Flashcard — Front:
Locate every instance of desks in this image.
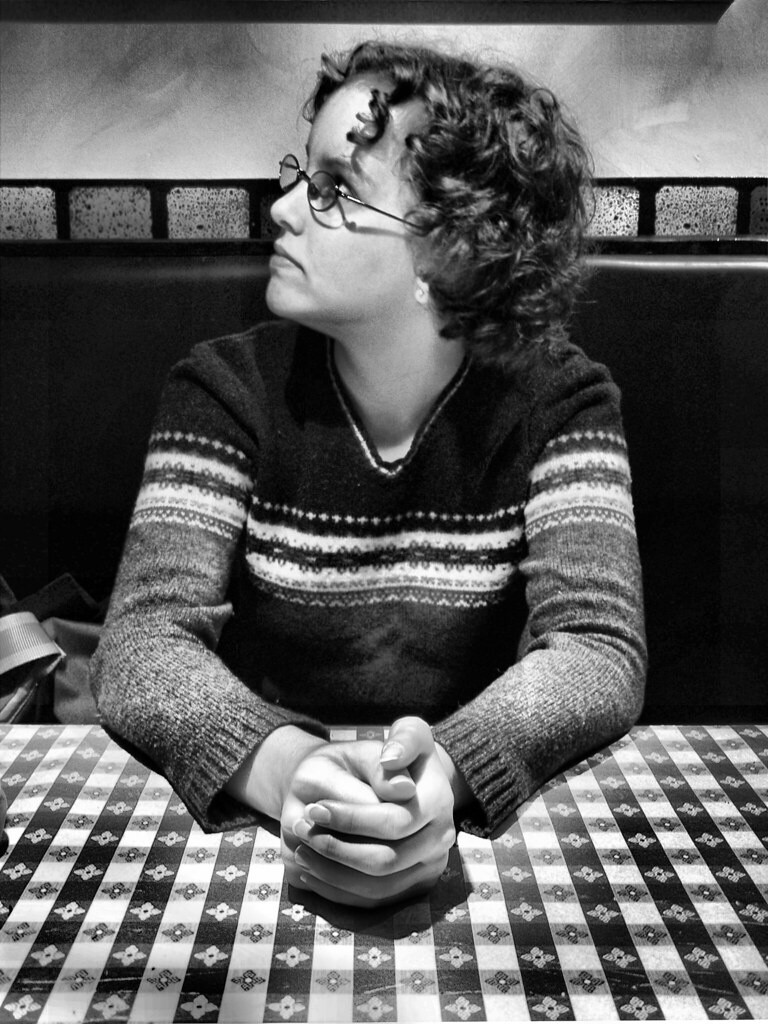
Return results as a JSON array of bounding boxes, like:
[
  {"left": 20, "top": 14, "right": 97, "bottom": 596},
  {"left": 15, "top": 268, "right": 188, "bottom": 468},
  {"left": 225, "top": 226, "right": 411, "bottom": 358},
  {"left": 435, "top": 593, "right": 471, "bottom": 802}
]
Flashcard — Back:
[{"left": 1, "top": 724, "right": 768, "bottom": 1021}]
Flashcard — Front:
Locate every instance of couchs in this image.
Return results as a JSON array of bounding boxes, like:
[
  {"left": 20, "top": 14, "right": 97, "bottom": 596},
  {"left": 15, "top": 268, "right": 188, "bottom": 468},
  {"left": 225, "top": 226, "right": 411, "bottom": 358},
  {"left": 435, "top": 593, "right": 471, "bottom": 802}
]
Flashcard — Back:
[{"left": 0, "top": 236, "right": 768, "bottom": 722}]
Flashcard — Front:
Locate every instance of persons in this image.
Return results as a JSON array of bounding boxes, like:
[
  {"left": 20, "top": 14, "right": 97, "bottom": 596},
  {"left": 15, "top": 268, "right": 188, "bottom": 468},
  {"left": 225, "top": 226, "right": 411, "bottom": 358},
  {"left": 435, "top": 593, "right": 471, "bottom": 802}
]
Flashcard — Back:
[{"left": 90, "top": 39, "right": 646, "bottom": 913}]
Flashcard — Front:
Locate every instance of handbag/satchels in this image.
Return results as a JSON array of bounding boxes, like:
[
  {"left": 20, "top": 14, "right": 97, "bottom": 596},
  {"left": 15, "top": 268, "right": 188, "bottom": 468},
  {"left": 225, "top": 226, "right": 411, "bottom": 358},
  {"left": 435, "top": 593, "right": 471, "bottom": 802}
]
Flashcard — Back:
[{"left": 0, "top": 570, "right": 106, "bottom": 725}]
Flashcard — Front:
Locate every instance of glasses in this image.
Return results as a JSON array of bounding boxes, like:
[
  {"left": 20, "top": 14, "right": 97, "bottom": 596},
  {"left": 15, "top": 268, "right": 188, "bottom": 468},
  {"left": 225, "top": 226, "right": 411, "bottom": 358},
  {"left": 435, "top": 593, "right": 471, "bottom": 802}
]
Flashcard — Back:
[{"left": 276, "top": 153, "right": 438, "bottom": 237}]
